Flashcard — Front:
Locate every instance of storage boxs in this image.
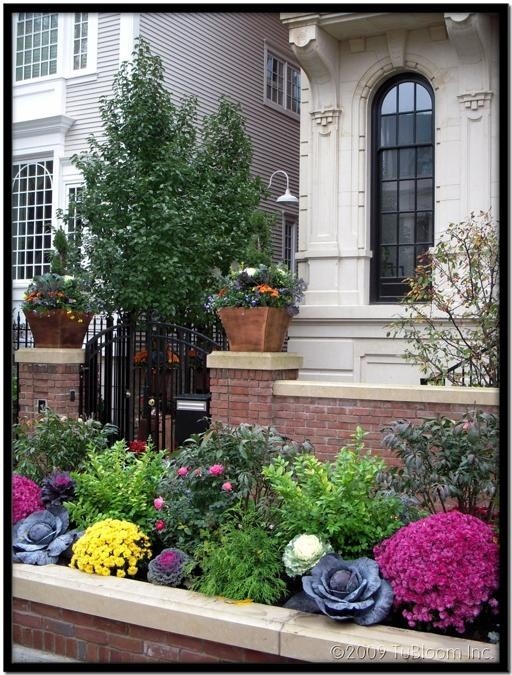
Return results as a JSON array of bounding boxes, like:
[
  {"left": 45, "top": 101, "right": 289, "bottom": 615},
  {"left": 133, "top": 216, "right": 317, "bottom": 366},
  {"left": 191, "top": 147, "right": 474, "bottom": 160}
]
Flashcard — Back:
[{"left": 173, "top": 393, "right": 211, "bottom": 447}]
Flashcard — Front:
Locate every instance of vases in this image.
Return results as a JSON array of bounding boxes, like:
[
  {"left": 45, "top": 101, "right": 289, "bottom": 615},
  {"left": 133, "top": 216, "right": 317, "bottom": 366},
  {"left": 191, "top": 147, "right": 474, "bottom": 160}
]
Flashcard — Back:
[
  {"left": 214, "top": 307, "right": 290, "bottom": 352},
  {"left": 23, "top": 308, "right": 94, "bottom": 348}
]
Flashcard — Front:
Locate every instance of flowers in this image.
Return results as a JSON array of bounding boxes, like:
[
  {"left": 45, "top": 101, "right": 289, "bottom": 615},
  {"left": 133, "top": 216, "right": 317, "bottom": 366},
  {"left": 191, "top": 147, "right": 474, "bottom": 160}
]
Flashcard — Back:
[
  {"left": 20, "top": 273, "right": 102, "bottom": 323},
  {"left": 203, "top": 259, "right": 310, "bottom": 317}
]
